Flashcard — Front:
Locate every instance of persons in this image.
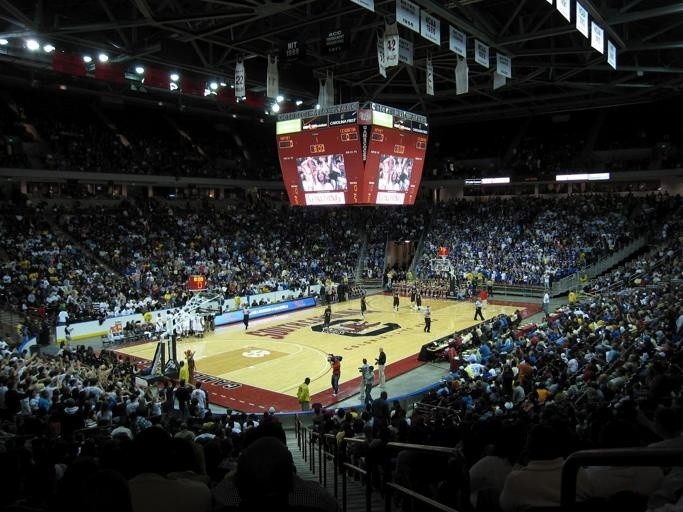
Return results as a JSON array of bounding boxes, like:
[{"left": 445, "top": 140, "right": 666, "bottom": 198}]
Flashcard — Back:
[{"left": 1, "top": 153, "right": 682, "bottom": 512}]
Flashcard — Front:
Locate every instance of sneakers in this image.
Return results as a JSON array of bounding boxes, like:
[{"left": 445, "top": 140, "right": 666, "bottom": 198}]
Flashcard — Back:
[
  {"left": 332, "top": 389, "right": 339, "bottom": 397},
  {"left": 378, "top": 384, "right": 385, "bottom": 388}
]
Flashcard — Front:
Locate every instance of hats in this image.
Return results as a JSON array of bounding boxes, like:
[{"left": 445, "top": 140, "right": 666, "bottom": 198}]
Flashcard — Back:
[{"left": 269, "top": 407, "right": 275, "bottom": 413}]
